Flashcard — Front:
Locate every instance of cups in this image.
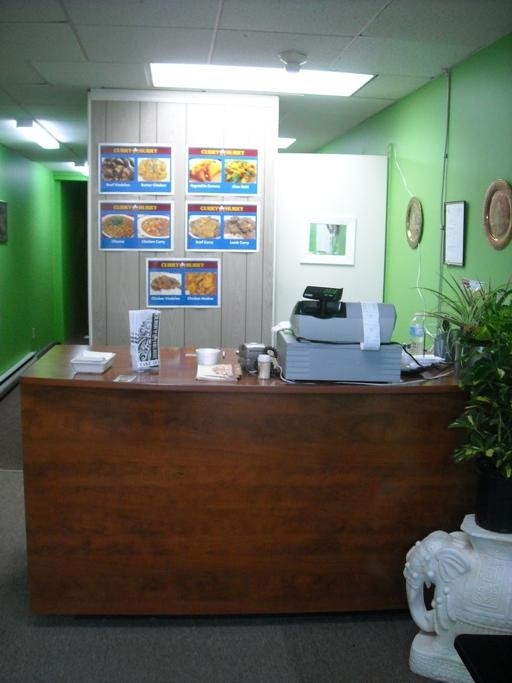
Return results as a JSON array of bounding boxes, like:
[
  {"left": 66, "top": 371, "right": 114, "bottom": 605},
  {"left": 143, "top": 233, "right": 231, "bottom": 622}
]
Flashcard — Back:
[
  {"left": 433, "top": 332, "right": 456, "bottom": 370},
  {"left": 195, "top": 347, "right": 221, "bottom": 364},
  {"left": 257, "top": 353, "right": 272, "bottom": 379}
]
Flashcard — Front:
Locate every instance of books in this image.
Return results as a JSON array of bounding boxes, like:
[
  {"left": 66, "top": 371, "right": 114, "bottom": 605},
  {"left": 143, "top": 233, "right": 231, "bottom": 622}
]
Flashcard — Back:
[{"left": 196, "top": 362, "right": 242, "bottom": 381}]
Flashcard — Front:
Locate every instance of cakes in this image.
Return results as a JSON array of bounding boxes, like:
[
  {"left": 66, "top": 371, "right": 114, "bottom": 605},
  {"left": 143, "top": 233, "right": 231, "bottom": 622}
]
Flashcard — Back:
[{"left": 190, "top": 217, "right": 220, "bottom": 239}]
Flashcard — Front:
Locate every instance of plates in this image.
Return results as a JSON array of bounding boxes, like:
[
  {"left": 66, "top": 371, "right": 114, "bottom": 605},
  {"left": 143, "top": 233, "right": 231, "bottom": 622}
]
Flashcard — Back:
[
  {"left": 188, "top": 215, "right": 222, "bottom": 240},
  {"left": 101, "top": 213, "right": 135, "bottom": 238},
  {"left": 405, "top": 196, "right": 423, "bottom": 248},
  {"left": 137, "top": 214, "right": 170, "bottom": 239},
  {"left": 482, "top": 178, "right": 512, "bottom": 248},
  {"left": 70, "top": 350, "right": 117, "bottom": 373}
]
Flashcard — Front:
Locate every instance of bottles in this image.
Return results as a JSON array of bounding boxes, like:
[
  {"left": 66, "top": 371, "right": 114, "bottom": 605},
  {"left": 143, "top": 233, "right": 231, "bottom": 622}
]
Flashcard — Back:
[{"left": 408, "top": 313, "right": 426, "bottom": 360}]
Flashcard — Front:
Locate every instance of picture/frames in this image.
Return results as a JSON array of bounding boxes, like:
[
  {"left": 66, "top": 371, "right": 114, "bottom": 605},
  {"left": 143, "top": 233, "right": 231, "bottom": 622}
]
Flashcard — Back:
[
  {"left": 0, "top": 201, "right": 9, "bottom": 243},
  {"left": 444, "top": 201, "right": 466, "bottom": 267},
  {"left": 299, "top": 215, "right": 357, "bottom": 266}
]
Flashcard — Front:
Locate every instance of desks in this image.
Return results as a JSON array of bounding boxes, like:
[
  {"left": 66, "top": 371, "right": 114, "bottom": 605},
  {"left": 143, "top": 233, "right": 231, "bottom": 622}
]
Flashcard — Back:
[{"left": 454, "top": 634, "right": 512, "bottom": 683}]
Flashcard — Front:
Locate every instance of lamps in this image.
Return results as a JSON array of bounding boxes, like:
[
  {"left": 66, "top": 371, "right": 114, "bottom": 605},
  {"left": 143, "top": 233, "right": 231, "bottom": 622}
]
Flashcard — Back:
[
  {"left": 278, "top": 51, "right": 311, "bottom": 75},
  {"left": 15, "top": 119, "right": 60, "bottom": 149}
]
